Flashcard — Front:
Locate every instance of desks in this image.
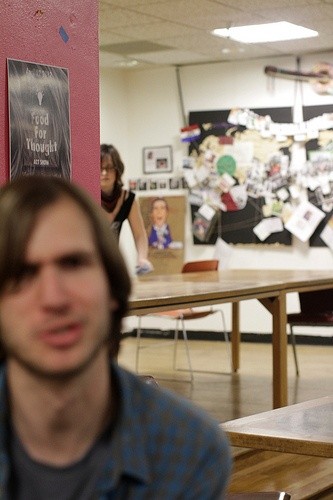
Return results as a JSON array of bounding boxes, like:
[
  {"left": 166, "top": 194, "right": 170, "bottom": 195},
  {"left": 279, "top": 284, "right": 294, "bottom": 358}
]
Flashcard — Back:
[
  {"left": 127, "top": 269, "right": 333, "bottom": 409},
  {"left": 218, "top": 395, "right": 333, "bottom": 459}
]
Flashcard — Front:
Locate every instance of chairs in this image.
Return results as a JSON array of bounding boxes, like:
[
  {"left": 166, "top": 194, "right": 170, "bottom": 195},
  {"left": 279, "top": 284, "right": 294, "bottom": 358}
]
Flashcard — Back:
[
  {"left": 135, "top": 259, "right": 236, "bottom": 383},
  {"left": 287, "top": 288, "right": 332, "bottom": 377}
]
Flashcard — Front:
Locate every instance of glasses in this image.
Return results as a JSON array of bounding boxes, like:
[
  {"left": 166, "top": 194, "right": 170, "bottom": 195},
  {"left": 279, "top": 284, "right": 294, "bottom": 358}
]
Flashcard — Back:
[{"left": 101, "top": 164, "right": 117, "bottom": 174}]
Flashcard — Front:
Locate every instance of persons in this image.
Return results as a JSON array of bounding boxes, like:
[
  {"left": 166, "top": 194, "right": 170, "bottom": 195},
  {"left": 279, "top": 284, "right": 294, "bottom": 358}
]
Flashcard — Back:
[
  {"left": 0, "top": 175, "right": 232, "bottom": 500},
  {"left": 147, "top": 198, "right": 183, "bottom": 249},
  {"left": 100, "top": 142, "right": 155, "bottom": 274}
]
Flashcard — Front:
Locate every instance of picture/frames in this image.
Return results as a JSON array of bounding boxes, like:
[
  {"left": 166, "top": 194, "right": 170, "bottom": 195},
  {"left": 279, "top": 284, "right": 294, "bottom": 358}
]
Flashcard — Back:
[{"left": 143, "top": 146, "right": 173, "bottom": 174}]
